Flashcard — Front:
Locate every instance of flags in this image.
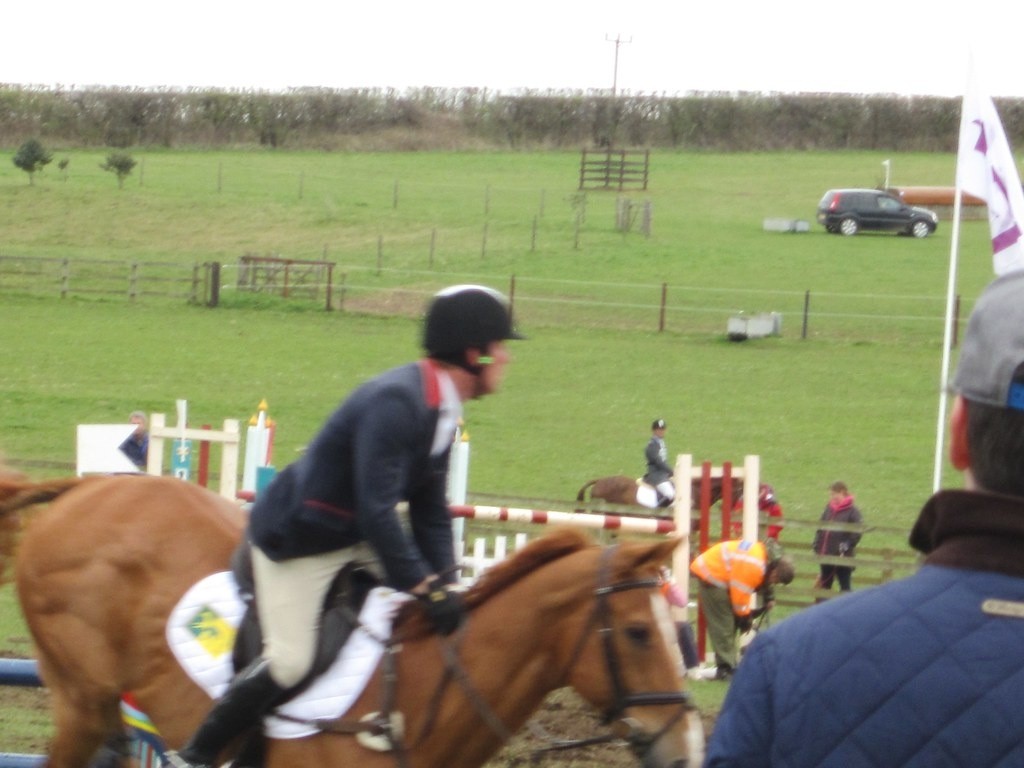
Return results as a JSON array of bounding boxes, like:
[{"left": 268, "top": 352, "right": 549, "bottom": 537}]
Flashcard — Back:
[{"left": 959, "top": 69, "right": 1024, "bottom": 277}]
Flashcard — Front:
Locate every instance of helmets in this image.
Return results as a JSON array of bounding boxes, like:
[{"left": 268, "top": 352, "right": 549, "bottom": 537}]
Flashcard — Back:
[
  {"left": 653, "top": 419, "right": 666, "bottom": 429},
  {"left": 421, "top": 286, "right": 526, "bottom": 376}
]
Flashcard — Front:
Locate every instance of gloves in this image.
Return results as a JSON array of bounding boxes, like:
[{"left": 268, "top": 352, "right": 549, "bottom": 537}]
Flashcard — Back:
[{"left": 419, "top": 578, "right": 461, "bottom": 636}]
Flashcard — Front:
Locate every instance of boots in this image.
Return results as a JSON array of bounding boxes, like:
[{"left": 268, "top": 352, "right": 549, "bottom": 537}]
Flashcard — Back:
[
  {"left": 166, "top": 657, "right": 291, "bottom": 768},
  {"left": 657, "top": 496, "right": 674, "bottom": 507}
]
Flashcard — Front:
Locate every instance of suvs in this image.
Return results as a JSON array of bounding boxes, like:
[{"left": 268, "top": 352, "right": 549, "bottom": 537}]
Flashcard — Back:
[{"left": 817, "top": 189, "right": 939, "bottom": 238}]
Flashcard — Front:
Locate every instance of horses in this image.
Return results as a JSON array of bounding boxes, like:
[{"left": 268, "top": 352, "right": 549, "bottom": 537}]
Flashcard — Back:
[
  {"left": 0, "top": 470, "right": 705, "bottom": 768},
  {"left": 577, "top": 476, "right": 744, "bottom": 527}
]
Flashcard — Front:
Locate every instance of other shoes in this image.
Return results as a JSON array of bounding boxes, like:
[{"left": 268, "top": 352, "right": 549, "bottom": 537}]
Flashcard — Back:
[{"left": 715, "top": 668, "right": 737, "bottom": 679}]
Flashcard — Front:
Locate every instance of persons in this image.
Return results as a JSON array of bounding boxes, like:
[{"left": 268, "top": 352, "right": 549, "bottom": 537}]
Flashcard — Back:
[
  {"left": 645, "top": 420, "right": 675, "bottom": 510},
  {"left": 160, "top": 284, "right": 524, "bottom": 768},
  {"left": 690, "top": 537, "right": 794, "bottom": 679},
  {"left": 812, "top": 481, "right": 865, "bottom": 603},
  {"left": 702, "top": 271, "right": 1023, "bottom": 768},
  {"left": 755, "top": 485, "right": 785, "bottom": 541}
]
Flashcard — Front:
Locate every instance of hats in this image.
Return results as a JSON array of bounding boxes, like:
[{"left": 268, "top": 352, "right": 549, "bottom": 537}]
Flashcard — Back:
[{"left": 947, "top": 268, "right": 1024, "bottom": 410}]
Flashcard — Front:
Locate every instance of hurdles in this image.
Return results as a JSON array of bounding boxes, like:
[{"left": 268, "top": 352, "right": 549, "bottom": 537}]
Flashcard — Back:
[
  {"left": 132, "top": 409, "right": 763, "bottom": 681},
  {"left": 234, "top": 396, "right": 695, "bottom": 624}
]
